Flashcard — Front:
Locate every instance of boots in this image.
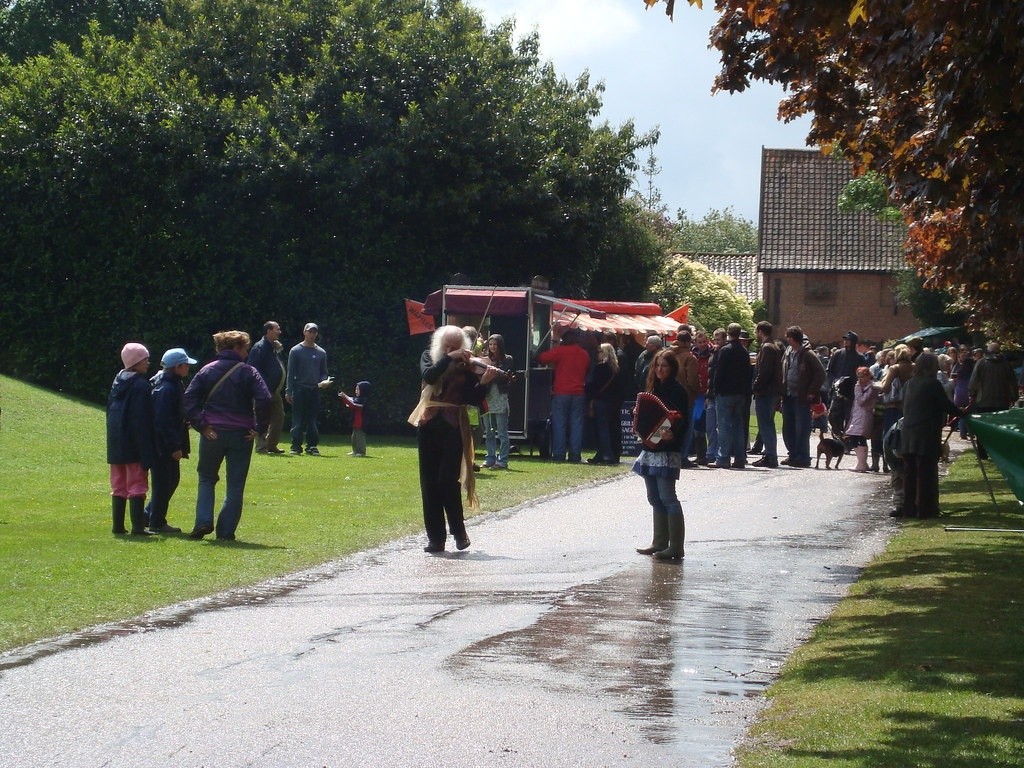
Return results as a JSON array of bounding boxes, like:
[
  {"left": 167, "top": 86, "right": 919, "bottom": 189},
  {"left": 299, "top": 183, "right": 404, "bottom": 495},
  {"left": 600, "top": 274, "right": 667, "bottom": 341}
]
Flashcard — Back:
[
  {"left": 636, "top": 511, "right": 670, "bottom": 556},
  {"left": 655, "top": 514, "right": 684, "bottom": 560},
  {"left": 127, "top": 494, "right": 154, "bottom": 535},
  {"left": 112, "top": 494, "right": 128, "bottom": 534},
  {"left": 848, "top": 447, "right": 870, "bottom": 473}
]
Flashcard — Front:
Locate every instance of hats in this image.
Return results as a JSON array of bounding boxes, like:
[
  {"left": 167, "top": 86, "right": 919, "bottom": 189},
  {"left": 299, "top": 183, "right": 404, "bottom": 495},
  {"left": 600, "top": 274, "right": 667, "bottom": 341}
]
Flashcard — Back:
[
  {"left": 161, "top": 347, "right": 198, "bottom": 367},
  {"left": 842, "top": 333, "right": 851, "bottom": 339},
  {"left": 304, "top": 323, "right": 318, "bottom": 332},
  {"left": 739, "top": 331, "right": 755, "bottom": 341},
  {"left": 121, "top": 343, "right": 150, "bottom": 370}
]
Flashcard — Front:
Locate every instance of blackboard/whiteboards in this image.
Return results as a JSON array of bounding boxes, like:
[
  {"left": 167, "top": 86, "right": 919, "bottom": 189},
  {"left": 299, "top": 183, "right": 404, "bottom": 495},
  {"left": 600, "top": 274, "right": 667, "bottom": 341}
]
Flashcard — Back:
[{"left": 619, "top": 402, "right": 644, "bottom": 457}]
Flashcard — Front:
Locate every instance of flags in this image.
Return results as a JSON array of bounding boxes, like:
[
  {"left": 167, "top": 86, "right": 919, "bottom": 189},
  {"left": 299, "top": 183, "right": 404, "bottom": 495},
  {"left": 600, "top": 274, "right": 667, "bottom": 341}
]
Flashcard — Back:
[
  {"left": 405, "top": 299, "right": 435, "bottom": 335},
  {"left": 665, "top": 305, "right": 688, "bottom": 341}
]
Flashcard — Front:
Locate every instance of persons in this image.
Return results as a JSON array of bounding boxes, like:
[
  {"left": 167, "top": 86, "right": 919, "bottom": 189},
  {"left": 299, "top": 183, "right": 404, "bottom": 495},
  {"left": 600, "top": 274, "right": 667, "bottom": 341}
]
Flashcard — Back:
[
  {"left": 462, "top": 326, "right": 480, "bottom": 472},
  {"left": 150, "top": 348, "right": 197, "bottom": 531},
  {"left": 185, "top": 332, "right": 272, "bottom": 542},
  {"left": 407, "top": 324, "right": 497, "bottom": 551},
  {"left": 480, "top": 334, "right": 513, "bottom": 469},
  {"left": 883, "top": 415, "right": 949, "bottom": 513},
  {"left": 903, "top": 352, "right": 968, "bottom": 511},
  {"left": 543, "top": 332, "right": 590, "bottom": 463},
  {"left": 601, "top": 332, "right": 625, "bottom": 366},
  {"left": 631, "top": 348, "right": 689, "bottom": 559},
  {"left": 285, "top": 323, "right": 328, "bottom": 456},
  {"left": 588, "top": 343, "right": 619, "bottom": 464},
  {"left": 338, "top": 380, "right": 371, "bottom": 457},
  {"left": 105, "top": 342, "right": 158, "bottom": 535},
  {"left": 624, "top": 320, "right": 1016, "bottom": 471},
  {"left": 249, "top": 321, "right": 285, "bottom": 453}
]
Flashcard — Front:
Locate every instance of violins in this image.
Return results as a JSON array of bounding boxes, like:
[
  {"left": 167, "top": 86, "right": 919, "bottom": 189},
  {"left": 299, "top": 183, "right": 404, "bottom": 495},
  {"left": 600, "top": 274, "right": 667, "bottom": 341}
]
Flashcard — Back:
[{"left": 460, "top": 353, "right": 518, "bottom": 384}]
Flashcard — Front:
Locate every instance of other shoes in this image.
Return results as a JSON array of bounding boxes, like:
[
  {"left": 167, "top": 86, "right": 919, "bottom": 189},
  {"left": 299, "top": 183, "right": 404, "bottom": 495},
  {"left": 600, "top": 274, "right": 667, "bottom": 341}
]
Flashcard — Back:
[
  {"left": 305, "top": 445, "right": 321, "bottom": 457},
  {"left": 479, "top": 463, "right": 494, "bottom": 469},
  {"left": 149, "top": 521, "right": 182, "bottom": 532},
  {"left": 424, "top": 539, "right": 444, "bottom": 551},
  {"left": 489, "top": 463, "right": 503, "bottom": 470},
  {"left": 891, "top": 506, "right": 909, "bottom": 517},
  {"left": 188, "top": 522, "right": 213, "bottom": 539},
  {"left": 682, "top": 445, "right": 812, "bottom": 468},
  {"left": 587, "top": 457, "right": 598, "bottom": 463},
  {"left": 455, "top": 530, "right": 470, "bottom": 549},
  {"left": 267, "top": 447, "right": 286, "bottom": 454},
  {"left": 290, "top": 449, "right": 299, "bottom": 455},
  {"left": 347, "top": 450, "right": 365, "bottom": 457}
]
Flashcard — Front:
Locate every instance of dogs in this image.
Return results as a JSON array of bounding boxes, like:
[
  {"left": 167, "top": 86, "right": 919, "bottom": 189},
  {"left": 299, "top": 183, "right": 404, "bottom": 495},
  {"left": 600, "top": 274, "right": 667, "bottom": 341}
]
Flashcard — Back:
[{"left": 815, "top": 427, "right": 850, "bottom": 469}]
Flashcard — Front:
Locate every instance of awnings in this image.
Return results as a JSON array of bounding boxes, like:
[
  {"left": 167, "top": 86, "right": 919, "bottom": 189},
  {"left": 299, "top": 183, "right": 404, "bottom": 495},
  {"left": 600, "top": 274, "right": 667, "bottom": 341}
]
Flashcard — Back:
[
  {"left": 553, "top": 315, "right": 696, "bottom": 336},
  {"left": 900, "top": 326, "right": 959, "bottom": 343}
]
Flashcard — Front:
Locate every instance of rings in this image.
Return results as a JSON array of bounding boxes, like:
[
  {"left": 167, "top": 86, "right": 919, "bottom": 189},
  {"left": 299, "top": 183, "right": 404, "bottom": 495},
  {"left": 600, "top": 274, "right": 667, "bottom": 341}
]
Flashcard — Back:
[{"left": 210, "top": 432, "right": 212, "bottom": 434}]
